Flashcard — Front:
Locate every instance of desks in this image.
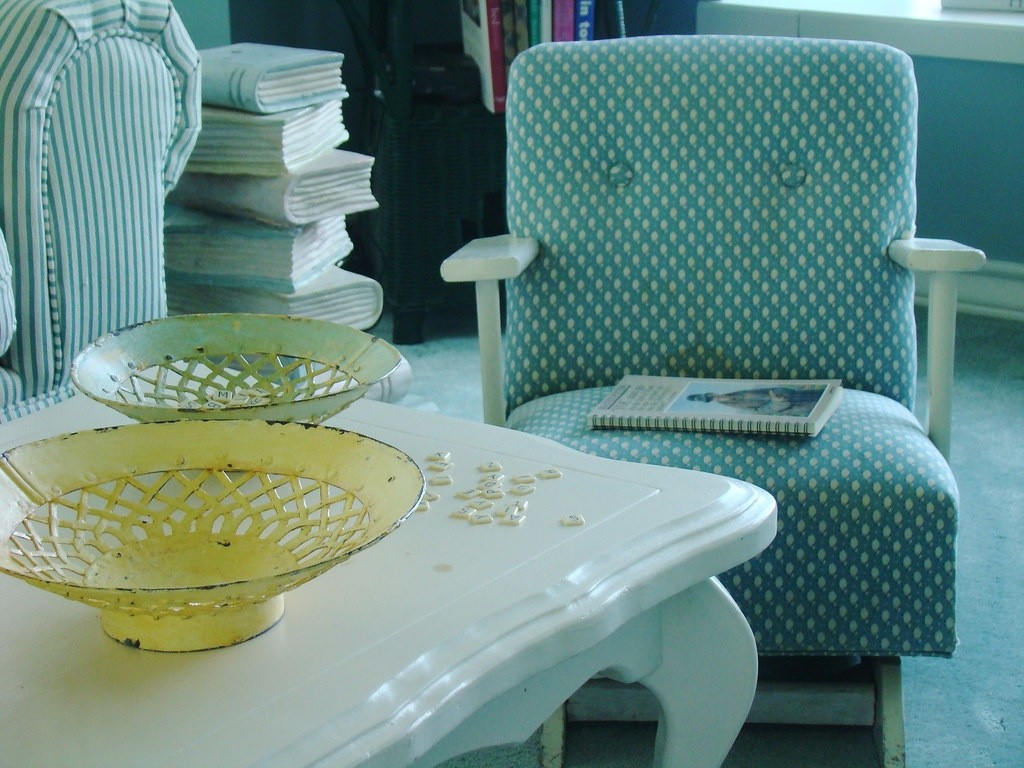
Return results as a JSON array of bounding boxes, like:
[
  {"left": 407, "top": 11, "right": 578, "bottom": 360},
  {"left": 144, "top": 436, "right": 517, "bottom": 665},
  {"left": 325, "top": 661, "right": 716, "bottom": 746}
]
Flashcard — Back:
[{"left": 0, "top": 360, "right": 779, "bottom": 768}]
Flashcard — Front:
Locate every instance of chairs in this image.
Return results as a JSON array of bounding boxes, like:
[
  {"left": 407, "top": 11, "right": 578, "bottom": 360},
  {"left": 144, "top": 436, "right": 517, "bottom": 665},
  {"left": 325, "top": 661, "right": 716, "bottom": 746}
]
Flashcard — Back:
[{"left": 440, "top": 34, "right": 987, "bottom": 767}]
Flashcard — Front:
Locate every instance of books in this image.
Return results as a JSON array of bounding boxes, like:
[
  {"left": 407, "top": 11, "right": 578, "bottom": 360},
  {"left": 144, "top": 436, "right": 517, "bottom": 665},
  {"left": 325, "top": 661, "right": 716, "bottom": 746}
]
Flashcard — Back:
[
  {"left": 461, "top": 0, "right": 595, "bottom": 114},
  {"left": 587, "top": 375, "right": 844, "bottom": 437},
  {"left": 161, "top": 42, "right": 435, "bottom": 410},
  {"left": 941, "top": 1, "right": 1024, "bottom": 12}
]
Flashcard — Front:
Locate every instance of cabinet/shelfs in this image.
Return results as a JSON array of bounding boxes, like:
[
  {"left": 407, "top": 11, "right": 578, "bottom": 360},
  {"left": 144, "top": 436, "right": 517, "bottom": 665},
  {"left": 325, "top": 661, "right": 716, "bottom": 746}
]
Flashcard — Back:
[{"left": 694, "top": 0, "right": 1024, "bottom": 320}]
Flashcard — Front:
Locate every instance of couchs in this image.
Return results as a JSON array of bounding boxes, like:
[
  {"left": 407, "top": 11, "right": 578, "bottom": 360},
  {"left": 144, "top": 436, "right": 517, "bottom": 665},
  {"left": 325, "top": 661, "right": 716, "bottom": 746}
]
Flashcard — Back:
[{"left": 0, "top": 0, "right": 203, "bottom": 423}]
